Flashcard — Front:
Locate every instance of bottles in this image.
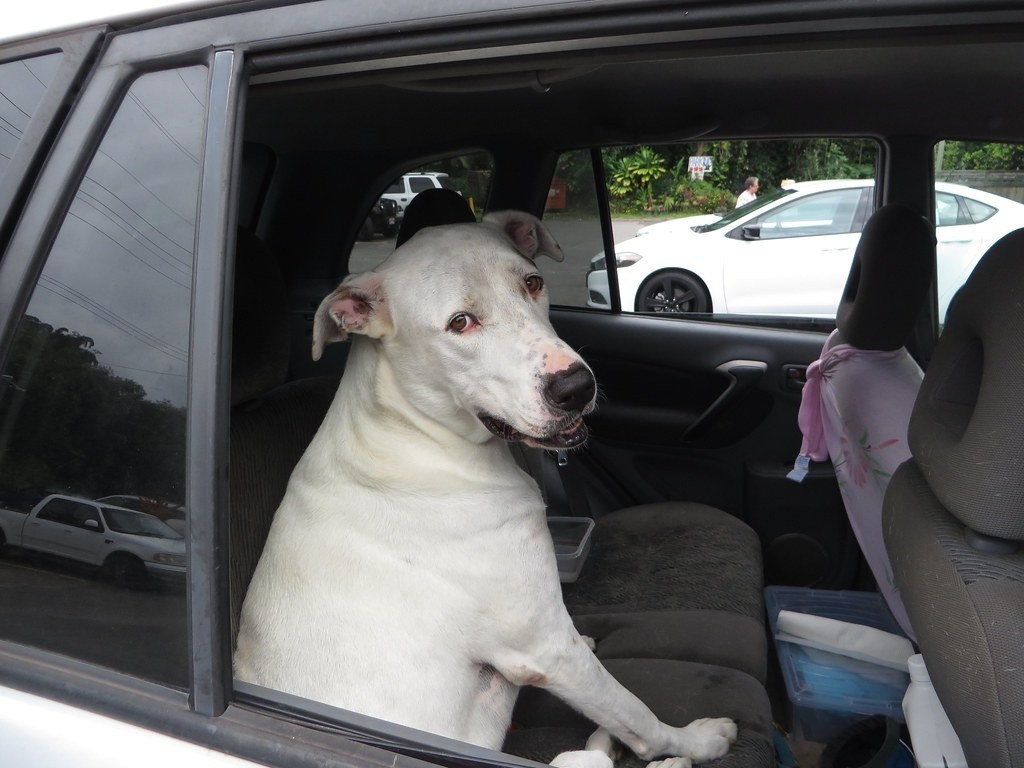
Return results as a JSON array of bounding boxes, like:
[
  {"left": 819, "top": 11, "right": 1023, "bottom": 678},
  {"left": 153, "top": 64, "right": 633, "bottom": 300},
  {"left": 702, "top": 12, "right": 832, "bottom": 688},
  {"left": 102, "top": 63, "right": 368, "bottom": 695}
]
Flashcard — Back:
[{"left": 902, "top": 654, "right": 969, "bottom": 768}]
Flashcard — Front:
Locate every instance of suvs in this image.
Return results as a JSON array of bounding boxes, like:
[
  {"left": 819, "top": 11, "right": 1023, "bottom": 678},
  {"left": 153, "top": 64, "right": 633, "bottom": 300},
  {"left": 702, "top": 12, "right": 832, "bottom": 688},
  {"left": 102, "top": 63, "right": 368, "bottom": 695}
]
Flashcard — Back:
[
  {"left": 355, "top": 170, "right": 466, "bottom": 243},
  {"left": 0, "top": 1, "right": 1024, "bottom": 768}
]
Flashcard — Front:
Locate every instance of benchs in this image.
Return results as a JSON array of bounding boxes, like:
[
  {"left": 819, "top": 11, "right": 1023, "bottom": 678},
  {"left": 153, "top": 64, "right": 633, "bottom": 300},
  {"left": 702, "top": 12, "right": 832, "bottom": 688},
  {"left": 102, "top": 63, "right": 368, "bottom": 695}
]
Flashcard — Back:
[{"left": 230, "top": 191, "right": 774, "bottom": 768}]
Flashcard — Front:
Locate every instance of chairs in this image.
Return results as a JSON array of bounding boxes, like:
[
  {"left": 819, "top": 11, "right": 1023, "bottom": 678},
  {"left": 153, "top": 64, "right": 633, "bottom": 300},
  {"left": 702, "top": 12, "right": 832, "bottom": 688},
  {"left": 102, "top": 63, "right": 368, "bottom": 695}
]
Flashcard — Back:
[
  {"left": 882, "top": 227, "right": 1024, "bottom": 768},
  {"left": 796, "top": 205, "right": 941, "bottom": 648}
]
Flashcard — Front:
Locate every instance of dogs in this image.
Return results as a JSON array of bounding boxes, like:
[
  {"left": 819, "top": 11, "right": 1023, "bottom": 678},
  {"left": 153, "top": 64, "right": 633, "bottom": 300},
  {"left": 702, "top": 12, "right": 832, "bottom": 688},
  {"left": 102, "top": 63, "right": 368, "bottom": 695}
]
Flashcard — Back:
[{"left": 230, "top": 211, "right": 738, "bottom": 768}]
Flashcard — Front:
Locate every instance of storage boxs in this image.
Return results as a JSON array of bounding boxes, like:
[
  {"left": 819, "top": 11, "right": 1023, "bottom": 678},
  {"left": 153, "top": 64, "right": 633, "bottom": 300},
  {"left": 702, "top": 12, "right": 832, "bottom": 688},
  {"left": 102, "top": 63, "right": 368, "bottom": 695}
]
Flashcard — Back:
[{"left": 761, "top": 585, "right": 921, "bottom": 742}]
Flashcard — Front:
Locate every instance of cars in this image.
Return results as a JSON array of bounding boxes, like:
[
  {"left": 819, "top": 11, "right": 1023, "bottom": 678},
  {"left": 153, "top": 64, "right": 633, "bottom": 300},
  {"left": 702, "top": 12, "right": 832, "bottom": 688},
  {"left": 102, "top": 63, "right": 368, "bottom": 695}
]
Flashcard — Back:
[{"left": 586, "top": 178, "right": 1024, "bottom": 337}]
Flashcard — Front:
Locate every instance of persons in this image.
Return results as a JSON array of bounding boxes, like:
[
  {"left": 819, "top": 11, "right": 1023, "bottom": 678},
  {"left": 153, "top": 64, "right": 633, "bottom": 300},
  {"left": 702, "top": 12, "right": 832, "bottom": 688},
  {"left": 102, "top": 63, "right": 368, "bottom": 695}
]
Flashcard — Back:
[{"left": 735, "top": 177, "right": 759, "bottom": 209}]
